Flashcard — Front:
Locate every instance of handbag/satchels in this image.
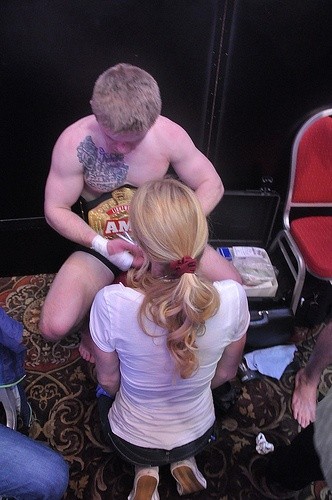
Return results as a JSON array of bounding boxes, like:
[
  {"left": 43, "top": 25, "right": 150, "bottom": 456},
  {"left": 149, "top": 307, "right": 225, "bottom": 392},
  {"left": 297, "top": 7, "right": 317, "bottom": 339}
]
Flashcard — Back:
[{"left": 278, "top": 280, "right": 332, "bottom": 328}]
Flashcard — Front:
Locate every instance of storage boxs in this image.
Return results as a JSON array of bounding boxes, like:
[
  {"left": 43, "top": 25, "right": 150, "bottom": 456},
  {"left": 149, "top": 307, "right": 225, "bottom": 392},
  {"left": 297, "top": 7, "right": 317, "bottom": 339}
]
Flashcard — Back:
[{"left": 202, "top": 187, "right": 295, "bottom": 351}]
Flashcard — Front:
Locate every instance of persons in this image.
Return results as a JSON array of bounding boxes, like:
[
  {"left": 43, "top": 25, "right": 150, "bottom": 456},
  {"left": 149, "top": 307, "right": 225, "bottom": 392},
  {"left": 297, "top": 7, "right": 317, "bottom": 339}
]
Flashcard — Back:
[
  {"left": 0, "top": 424, "right": 69, "bottom": 500},
  {"left": 89, "top": 178, "right": 251, "bottom": 500},
  {"left": 38, "top": 63, "right": 244, "bottom": 363},
  {"left": 265, "top": 320, "right": 332, "bottom": 493}
]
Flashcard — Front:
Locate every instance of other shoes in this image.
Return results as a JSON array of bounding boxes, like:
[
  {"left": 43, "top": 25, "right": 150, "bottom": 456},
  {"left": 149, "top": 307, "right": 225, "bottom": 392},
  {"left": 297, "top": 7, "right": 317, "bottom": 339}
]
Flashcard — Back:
[
  {"left": 238, "top": 445, "right": 271, "bottom": 496},
  {"left": 214, "top": 380, "right": 234, "bottom": 413}
]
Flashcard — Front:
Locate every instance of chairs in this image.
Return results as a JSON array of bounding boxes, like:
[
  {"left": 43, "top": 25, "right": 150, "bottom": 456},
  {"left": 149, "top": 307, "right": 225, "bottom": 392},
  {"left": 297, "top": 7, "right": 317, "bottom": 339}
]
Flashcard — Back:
[{"left": 269, "top": 109, "right": 332, "bottom": 318}]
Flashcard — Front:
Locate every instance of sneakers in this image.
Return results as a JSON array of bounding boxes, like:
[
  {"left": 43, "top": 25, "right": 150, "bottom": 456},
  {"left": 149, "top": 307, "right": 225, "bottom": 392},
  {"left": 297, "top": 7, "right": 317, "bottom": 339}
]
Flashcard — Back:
[
  {"left": 127, "top": 464, "right": 160, "bottom": 500},
  {"left": 170, "top": 456, "right": 207, "bottom": 495}
]
